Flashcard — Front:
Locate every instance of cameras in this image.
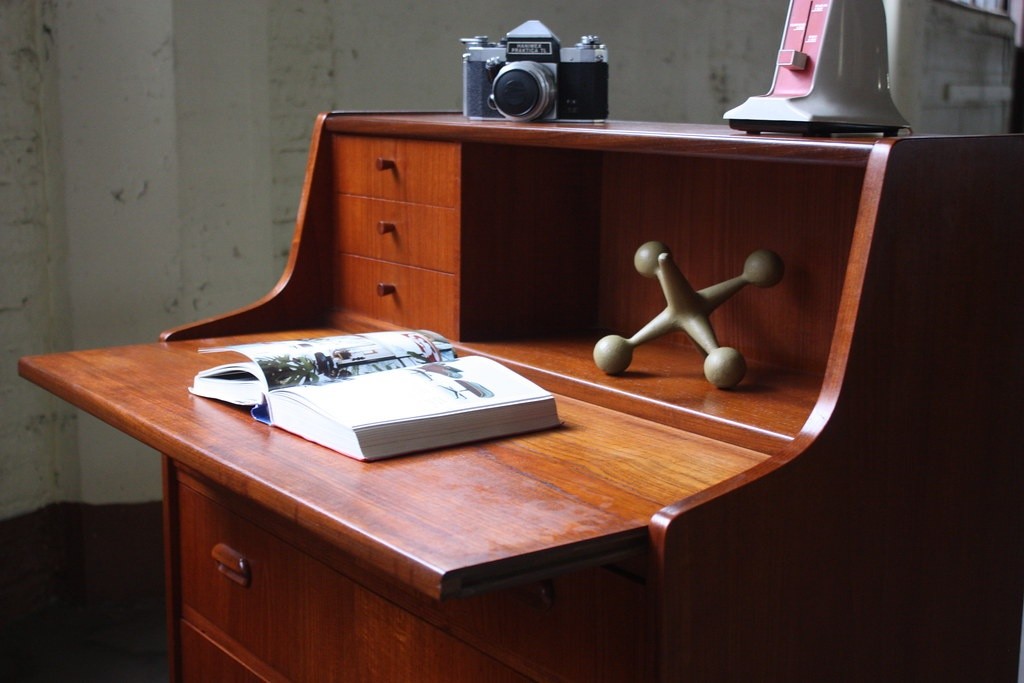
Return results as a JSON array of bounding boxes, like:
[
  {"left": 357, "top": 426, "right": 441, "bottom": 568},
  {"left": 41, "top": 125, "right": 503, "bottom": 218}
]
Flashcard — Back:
[{"left": 460, "top": 20, "right": 609, "bottom": 123}]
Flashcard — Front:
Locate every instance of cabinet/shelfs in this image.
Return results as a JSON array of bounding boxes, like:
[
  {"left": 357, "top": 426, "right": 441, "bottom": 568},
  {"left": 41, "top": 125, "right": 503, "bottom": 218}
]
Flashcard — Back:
[{"left": 16, "top": 106, "right": 1024, "bottom": 683}]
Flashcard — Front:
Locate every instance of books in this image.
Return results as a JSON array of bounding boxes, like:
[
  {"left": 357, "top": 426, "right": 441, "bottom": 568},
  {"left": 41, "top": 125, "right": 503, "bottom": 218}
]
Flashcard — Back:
[{"left": 188, "top": 330, "right": 565, "bottom": 462}]
